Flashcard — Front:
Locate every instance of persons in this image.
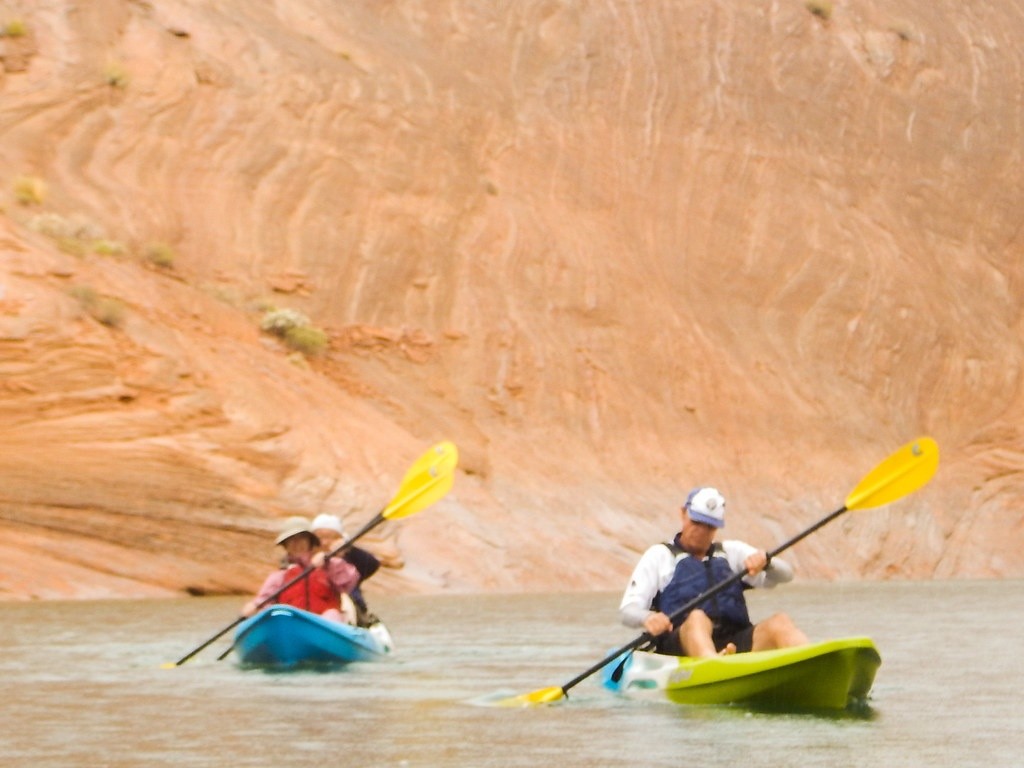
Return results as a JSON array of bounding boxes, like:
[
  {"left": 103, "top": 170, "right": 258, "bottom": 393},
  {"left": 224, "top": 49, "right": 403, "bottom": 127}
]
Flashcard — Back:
[
  {"left": 618, "top": 486, "right": 806, "bottom": 659},
  {"left": 237, "top": 510, "right": 406, "bottom": 629}
]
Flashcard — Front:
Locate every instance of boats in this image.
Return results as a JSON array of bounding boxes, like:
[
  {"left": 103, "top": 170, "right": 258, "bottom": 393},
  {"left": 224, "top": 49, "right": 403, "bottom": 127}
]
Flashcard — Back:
[
  {"left": 230, "top": 602, "right": 384, "bottom": 674},
  {"left": 602, "top": 635, "right": 884, "bottom": 715}
]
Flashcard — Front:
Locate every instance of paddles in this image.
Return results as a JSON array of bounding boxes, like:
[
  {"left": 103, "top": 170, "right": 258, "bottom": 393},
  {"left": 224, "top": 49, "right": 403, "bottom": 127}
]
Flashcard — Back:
[
  {"left": 499, "top": 435, "right": 940, "bottom": 711},
  {"left": 168, "top": 438, "right": 460, "bottom": 674}
]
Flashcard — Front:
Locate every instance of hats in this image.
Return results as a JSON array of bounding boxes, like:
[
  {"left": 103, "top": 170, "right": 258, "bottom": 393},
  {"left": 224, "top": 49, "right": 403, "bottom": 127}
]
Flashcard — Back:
[
  {"left": 686, "top": 487, "right": 725, "bottom": 528},
  {"left": 311, "top": 514, "right": 351, "bottom": 542},
  {"left": 274, "top": 516, "right": 321, "bottom": 547}
]
